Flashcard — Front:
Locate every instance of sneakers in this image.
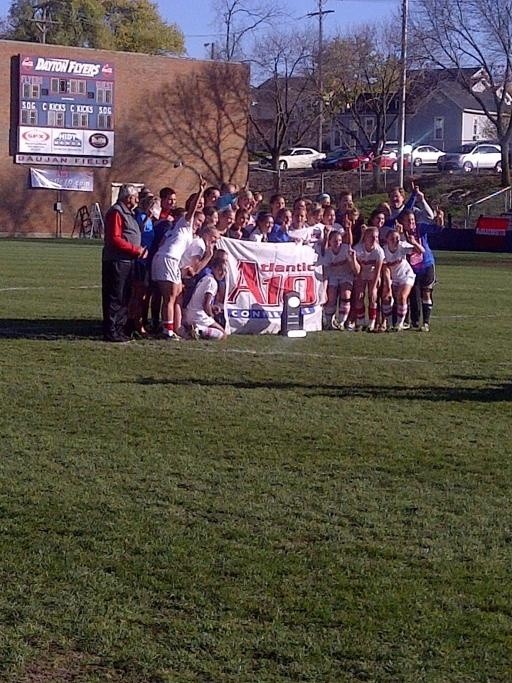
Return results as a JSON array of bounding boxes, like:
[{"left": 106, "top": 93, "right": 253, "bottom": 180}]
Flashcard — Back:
[
  {"left": 325, "top": 321, "right": 430, "bottom": 333},
  {"left": 192, "top": 321, "right": 200, "bottom": 339},
  {"left": 168, "top": 333, "right": 182, "bottom": 342}
]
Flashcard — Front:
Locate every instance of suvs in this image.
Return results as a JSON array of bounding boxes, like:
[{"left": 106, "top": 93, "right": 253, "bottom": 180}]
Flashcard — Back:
[
  {"left": 437, "top": 140, "right": 502, "bottom": 173},
  {"left": 380, "top": 140, "right": 411, "bottom": 163}
]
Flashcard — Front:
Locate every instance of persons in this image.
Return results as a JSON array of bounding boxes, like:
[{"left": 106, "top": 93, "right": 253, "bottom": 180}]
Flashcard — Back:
[{"left": 101, "top": 168, "right": 444, "bottom": 342}]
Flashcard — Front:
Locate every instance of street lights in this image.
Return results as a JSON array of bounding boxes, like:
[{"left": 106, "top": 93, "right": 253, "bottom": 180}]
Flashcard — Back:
[{"left": 204, "top": 39, "right": 215, "bottom": 59}]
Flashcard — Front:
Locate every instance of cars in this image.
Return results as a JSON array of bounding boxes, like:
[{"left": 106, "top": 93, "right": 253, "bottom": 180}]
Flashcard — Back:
[
  {"left": 318, "top": 145, "right": 351, "bottom": 168},
  {"left": 258, "top": 146, "right": 326, "bottom": 170},
  {"left": 409, "top": 144, "right": 447, "bottom": 166},
  {"left": 364, "top": 140, "right": 377, "bottom": 152},
  {"left": 336, "top": 144, "right": 408, "bottom": 172}
]
